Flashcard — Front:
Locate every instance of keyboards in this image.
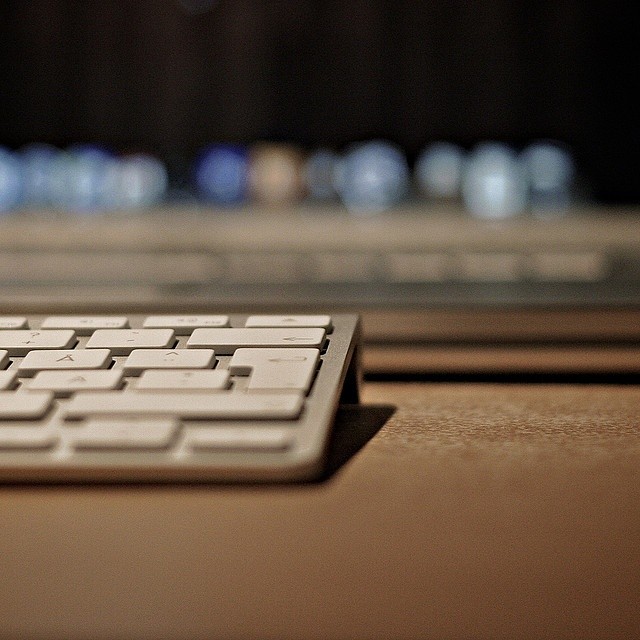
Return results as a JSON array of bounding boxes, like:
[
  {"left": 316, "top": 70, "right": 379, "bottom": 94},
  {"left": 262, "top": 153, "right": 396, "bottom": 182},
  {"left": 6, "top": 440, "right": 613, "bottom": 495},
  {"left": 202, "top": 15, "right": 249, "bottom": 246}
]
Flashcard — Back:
[{"left": 0, "top": 311, "right": 362, "bottom": 485}]
